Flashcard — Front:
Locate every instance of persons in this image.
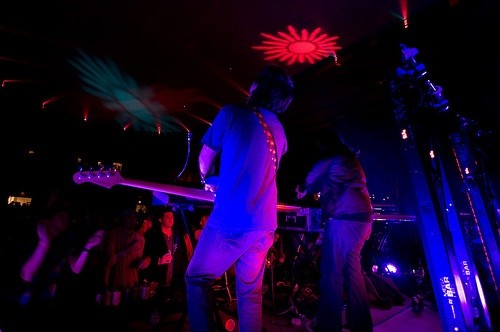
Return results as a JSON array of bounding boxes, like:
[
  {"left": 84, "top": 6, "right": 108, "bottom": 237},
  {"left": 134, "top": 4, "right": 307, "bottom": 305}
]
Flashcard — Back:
[
  {"left": 19, "top": 204, "right": 183, "bottom": 306},
  {"left": 185, "top": 66, "right": 374, "bottom": 332}
]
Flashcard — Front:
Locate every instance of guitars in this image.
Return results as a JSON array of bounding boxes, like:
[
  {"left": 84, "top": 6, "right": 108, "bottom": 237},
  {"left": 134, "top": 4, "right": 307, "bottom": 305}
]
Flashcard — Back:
[{"left": 71, "top": 161, "right": 215, "bottom": 203}]
